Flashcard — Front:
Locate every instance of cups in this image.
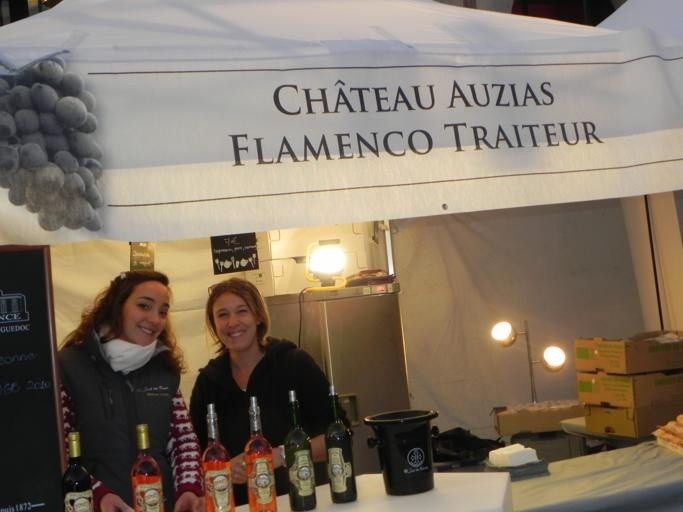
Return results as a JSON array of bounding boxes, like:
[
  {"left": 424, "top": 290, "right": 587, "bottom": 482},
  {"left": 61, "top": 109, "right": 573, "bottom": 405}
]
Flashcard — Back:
[{"left": 363, "top": 408, "right": 439, "bottom": 495}]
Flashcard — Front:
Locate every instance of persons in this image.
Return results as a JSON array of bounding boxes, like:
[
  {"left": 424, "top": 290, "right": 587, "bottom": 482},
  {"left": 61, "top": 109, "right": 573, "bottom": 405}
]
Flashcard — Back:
[
  {"left": 189, "top": 277, "right": 353, "bottom": 507},
  {"left": 56, "top": 268, "right": 205, "bottom": 511}
]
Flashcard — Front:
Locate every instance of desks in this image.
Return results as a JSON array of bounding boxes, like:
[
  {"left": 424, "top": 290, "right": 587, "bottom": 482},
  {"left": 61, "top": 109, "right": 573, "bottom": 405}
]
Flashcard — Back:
[
  {"left": 235, "top": 471, "right": 510, "bottom": 512},
  {"left": 561, "top": 416, "right": 607, "bottom": 458},
  {"left": 510, "top": 441, "right": 683, "bottom": 512}
]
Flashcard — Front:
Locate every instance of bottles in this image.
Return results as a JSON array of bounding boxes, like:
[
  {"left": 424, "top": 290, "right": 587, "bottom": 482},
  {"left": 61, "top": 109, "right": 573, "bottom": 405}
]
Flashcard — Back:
[
  {"left": 202, "top": 403, "right": 236, "bottom": 511},
  {"left": 325, "top": 385, "right": 358, "bottom": 503},
  {"left": 244, "top": 397, "right": 278, "bottom": 512},
  {"left": 131, "top": 422, "right": 164, "bottom": 512},
  {"left": 62, "top": 428, "right": 93, "bottom": 512},
  {"left": 283, "top": 389, "right": 316, "bottom": 512}
]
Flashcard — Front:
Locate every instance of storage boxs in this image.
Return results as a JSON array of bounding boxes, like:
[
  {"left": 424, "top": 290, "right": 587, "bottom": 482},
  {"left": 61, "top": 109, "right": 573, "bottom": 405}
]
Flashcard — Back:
[{"left": 574, "top": 330, "right": 683, "bottom": 438}]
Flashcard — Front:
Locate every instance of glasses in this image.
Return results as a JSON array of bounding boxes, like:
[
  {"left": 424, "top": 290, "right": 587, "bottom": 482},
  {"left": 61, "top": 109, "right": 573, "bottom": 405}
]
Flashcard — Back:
[{"left": 207, "top": 280, "right": 250, "bottom": 297}]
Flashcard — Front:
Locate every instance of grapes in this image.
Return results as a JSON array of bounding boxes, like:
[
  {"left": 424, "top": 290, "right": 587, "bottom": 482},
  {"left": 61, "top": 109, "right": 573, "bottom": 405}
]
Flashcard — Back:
[{"left": 0, "top": 51, "right": 104, "bottom": 233}]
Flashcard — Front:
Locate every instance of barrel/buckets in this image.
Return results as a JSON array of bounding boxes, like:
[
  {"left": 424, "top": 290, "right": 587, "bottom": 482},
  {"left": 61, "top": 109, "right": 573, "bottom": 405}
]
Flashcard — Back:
[{"left": 364, "top": 395, "right": 441, "bottom": 498}]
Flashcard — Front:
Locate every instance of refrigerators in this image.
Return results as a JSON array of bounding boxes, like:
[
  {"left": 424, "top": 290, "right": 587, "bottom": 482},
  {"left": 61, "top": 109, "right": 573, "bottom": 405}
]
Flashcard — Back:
[{"left": 263, "top": 282, "right": 410, "bottom": 476}]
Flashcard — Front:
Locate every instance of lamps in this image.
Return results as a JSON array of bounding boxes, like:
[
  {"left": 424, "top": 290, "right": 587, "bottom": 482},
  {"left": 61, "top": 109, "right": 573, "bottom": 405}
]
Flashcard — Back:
[
  {"left": 306, "top": 239, "right": 347, "bottom": 293},
  {"left": 491, "top": 320, "right": 566, "bottom": 404}
]
